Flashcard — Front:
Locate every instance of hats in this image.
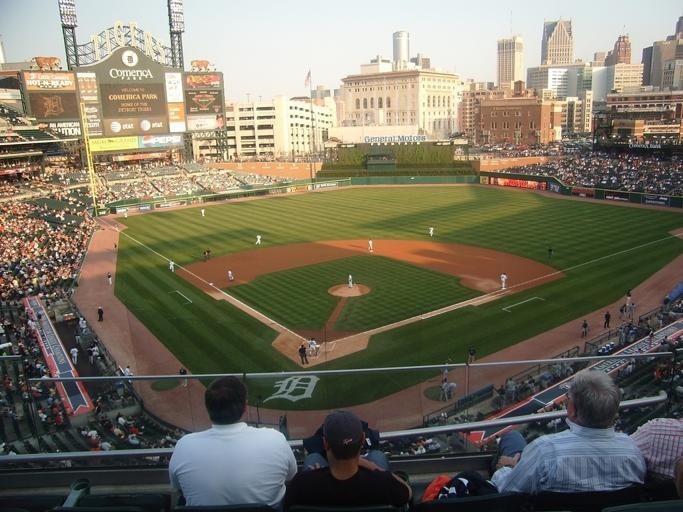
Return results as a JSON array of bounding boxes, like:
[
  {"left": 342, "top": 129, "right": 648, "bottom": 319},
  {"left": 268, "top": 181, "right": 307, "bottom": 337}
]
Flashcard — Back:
[{"left": 323, "top": 410, "right": 364, "bottom": 446}]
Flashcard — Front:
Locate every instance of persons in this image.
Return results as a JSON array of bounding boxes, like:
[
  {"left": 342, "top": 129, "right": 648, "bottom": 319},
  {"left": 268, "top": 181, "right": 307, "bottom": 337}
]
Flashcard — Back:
[
  {"left": 255, "top": 234, "right": 261, "bottom": 245},
  {"left": 202, "top": 248, "right": 210, "bottom": 257},
  {"left": 367, "top": 239, "right": 372, "bottom": 250},
  {"left": 168, "top": 375, "right": 299, "bottom": 512},
  {"left": 298, "top": 344, "right": 309, "bottom": 365},
  {"left": 460, "top": 126, "right": 682, "bottom": 201},
  {"left": 348, "top": 273, "right": 353, "bottom": 287},
  {"left": 548, "top": 247, "right": 552, "bottom": 257},
  {"left": 308, "top": 337, "right": 318, "bottom": 356},
  {"left": 200, "top": 208, "right": 205, "bottom": 217},
  {"left": 0, "top": 101, "right": 66, "bottom": 150},
  {"left": 500, "top": 272, "right": 507, "bottom": 289},
  {"left": 283, "top": 408, "right": 416, "bottom": 512},
  {"left": 428, "top": 226, "right": 433, "bottom": 236},
  {"left": 227, "top": 270, "right": 233, "bottom": 281},
  {"left": 288, "top": 434, "right": 424, "bottom": 466},
  {"left": 625, "top": 289, "right": 632, "bottom": 301}
]
